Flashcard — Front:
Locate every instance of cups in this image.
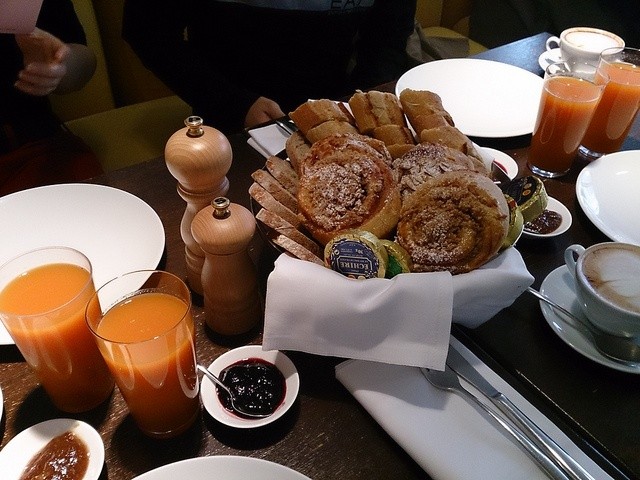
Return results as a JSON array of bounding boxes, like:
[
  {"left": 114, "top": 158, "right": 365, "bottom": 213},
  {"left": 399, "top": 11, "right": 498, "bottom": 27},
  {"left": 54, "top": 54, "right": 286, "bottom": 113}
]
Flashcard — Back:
[
  {"left": 577, "top": 47, "right": 640, "bottom": 159},
  {"left": 565, "top": 242, "right": 640, "bottom": 339},
  {"left": 85, "top": 269, "right": 201, "bottom": 439},
  {"left": 1, "top": 247, "right": 115, "bottom": 414},
  {"left": 526, "top": 62, "right": 608, "bottom": 177},
  {"left": 546, "top": 27, "right": 625, "bottom": 74}
]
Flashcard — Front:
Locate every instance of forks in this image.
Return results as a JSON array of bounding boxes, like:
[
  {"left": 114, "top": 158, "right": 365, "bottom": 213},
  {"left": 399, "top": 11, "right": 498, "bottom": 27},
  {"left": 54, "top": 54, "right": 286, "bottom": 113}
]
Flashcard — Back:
[{"left": 419, "top": 363, "right": 570, "bottom": 479}]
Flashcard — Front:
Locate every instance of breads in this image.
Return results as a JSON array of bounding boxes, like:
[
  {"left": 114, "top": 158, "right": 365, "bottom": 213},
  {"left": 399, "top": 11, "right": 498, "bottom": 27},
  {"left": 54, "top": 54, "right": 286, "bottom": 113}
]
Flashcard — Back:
[
  {"left": 392, "top": 140, "right": 474, "bottom": 206},
  {"left": 386, "top": 145, "right": 415, "bottom": 160},
  {"left": 421, "top": 127, "right": 472, "bottom": 154},
  {"left": 300, "top": 133, "right": 392, "bottom": 176},
  {"left": 288, "top": 99, "right": 355, "bottom": 136},
  {"left": 248, "top": 182, "right": 311, "bottom": 229},
  {"left": 306, "top": 120, "right": 359, "bottom": 144},
  {"left": 373, "top": 125, "right": 414, "bottom": 146},
  {"left": 298, "top": 150, "right": 401, "bottom": 246},
  {"left": 275, "top": 235, "right": 324, "bottom": 268},
  {"left": 265, "top": 155, "right": 315, "bottom": 195},
  {"left": 347, "top": 89, "right": 408, "bottom": 132},
  {"left": 285, "top": 129, "right": 310, "bottom": 174},
  {"left": 256, "top": 208, "right": 332, "bottom": 259},
  {"left": 397, "top": 170, "right": 511, "bottom": 274},
  {"left": 251, "top": 168, "right": 308, "bottom": 214},
  {"left": 399, "top": 88, "right": 455, "bottom": 139}
]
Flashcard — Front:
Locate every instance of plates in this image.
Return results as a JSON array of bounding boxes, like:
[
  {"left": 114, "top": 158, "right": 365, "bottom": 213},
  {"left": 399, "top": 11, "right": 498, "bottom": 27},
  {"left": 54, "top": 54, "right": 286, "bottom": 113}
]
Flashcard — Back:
[
  {"left": 202, "top": 344, "right": 300, "bottom": 430},
  {"left": 1, "top": 419, "right": 105, "bottom": 479},
  {"left": 539, "top": 262, "right": 640, "bottom": 374},
  {"left": 132, "top": 455, "right": 312, "bottom": 480},
  {"left": 1, "top": 184, "right": 165, "bottom": 346},
  {"left": 471, "top": 146, "right": 520, "bottom": 184},
  {"left": 522, "top": 196, "right": 573, "bottom": 237},
  {"left": 396, "top": 57, "right": 544, "bottom": 138},
  {"left": 576, "top": 149, "right": 640, "bottom": 245}
]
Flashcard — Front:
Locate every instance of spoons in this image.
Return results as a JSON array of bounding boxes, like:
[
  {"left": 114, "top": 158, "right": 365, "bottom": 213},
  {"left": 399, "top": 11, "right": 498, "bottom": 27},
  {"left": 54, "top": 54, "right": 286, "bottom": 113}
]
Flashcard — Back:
[
  {"left": 197, "top": 361, "right": 272, "bottom": 419},
  {"left": 527, "top": 287, "right": 640, "bottom": 363}
]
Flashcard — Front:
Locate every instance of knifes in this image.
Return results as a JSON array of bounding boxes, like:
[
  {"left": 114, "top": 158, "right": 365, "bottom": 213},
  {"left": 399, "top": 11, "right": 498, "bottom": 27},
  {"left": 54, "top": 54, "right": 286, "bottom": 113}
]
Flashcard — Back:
[{"left": 446, "top": 343, "right": 597, "bottom": 479}]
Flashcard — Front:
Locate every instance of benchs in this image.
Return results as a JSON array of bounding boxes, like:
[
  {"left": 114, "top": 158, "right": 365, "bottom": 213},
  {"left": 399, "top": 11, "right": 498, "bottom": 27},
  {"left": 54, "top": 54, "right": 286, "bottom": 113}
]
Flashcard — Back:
[{"left": 27, "top": 0, "right": 490, "bottom": 181}]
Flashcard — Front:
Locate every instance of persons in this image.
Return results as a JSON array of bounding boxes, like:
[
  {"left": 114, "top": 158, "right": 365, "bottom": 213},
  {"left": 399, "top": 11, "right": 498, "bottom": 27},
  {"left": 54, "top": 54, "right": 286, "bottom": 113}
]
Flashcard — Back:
[
  {"left": 3, "top": 0, "right": 99, "bottom": 119},
  {"left": 122, "top": 0, "right": 428, "bottom": 127}
]
